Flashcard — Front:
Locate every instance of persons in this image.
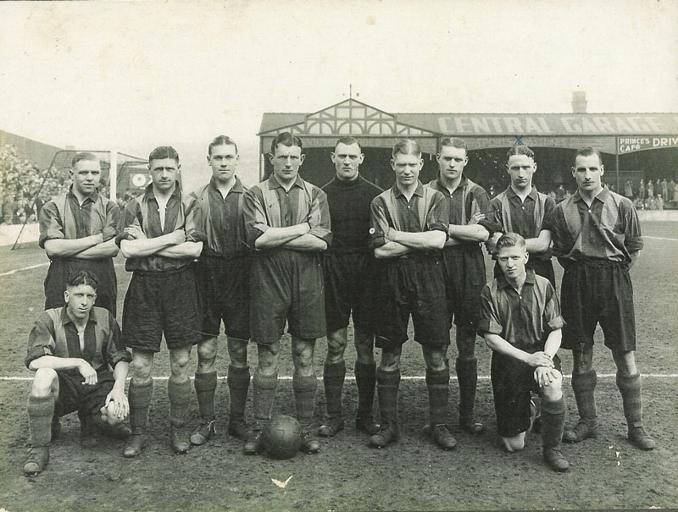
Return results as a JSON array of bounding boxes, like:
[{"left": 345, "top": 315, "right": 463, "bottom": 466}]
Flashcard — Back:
[
  {"left": 367, "top": 137, "right": 458, "bottom": 449},
  {"left": 484, "top": 145, "right": 556, "bottom": 288},
  {"left": 485, "top": 176, "right": 678, "bottom": 211},
  {"left": 319, "top": 135, "right": 385, "bottom": 437},
  {"left": 22, "top": 152, "right": 133, "bottom": 474},
  {"left": 242, "top": 131, "right": 321, "bottom": 456},
  {"left": 550, "top": 147, "right": 656, "bottom": 451},
  {"left": 476, "top": 231, "right": 571, "bottom": 472},
  {"left": 113, "top": 146, "right": 206, "bottom": 457},
  {"left": 191, "top": 133, "right": 254, "bottom": 446},
  {"left": 0, "top": 141, "right": 144, "bottom": 226},
  {"left": 424, "top": 136, "right": 491, "bottom": 435}
]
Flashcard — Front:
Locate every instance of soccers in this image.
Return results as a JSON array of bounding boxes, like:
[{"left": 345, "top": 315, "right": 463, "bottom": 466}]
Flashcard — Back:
[{"left": 264, "top": 415, "right": 302, "bottom": 458}]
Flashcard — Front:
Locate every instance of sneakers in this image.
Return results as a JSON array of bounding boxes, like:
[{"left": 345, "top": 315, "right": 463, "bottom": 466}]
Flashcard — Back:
[
  {"left": 627, "top": 423, "right": 656, "bottom": 450},
  {"left": 23, "top": 418, "right": 250, "bottom": 474},
  {"left": 562, "top": 416, "right": 598, "bottom": 442},
  {"left": 244, "top": 424, "right": 263, "bottom": 452},
  {"left": 356, "top": 410, "right": 382, "bottom": 434},
  {"left": 431, "top": 423, "right": 458, "bottom": 450},
  {"left": 318, "top": 413, "right": 344, "bottom": 436},
  {"left": 369, "top": 422, "right": 400, "bottom": 446},
  {"left": 460, "top": 407, "right": 485, "bottom": 434},
  {"left": 541, "top": 447, "right": 569, "bottom": 471},
  {"left": 301, "top": 423, "right": 320, "bottom": 454}
]
